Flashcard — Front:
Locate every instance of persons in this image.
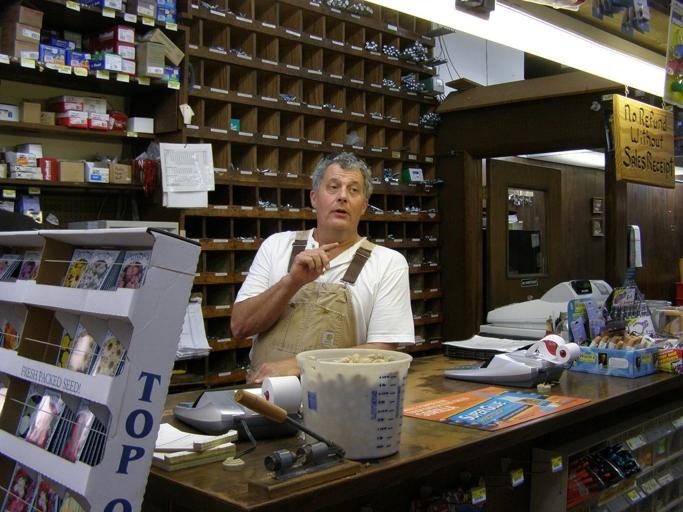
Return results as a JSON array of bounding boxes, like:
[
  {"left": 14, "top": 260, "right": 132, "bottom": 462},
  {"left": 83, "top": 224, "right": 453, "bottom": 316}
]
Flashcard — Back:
[{"left": 228, "top": 153, "right": 418, "bottom": 390}]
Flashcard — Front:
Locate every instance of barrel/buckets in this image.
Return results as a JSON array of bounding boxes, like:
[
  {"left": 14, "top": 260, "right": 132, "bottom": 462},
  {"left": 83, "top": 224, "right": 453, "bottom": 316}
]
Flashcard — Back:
[{"left": 296, "top": 346, "right": 413, "bottom": 463}]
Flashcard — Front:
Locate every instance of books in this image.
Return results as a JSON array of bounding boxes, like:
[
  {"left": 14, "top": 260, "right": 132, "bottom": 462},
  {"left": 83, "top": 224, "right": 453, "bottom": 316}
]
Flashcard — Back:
[{"left": 0, "top": 246, "right": 152, "bottom": 512}]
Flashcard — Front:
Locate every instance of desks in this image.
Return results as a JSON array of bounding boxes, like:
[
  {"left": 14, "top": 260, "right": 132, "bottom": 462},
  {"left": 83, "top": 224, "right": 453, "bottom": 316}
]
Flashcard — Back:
[{"left": 148, "top": 354, "right": 682, "bottom": 512}]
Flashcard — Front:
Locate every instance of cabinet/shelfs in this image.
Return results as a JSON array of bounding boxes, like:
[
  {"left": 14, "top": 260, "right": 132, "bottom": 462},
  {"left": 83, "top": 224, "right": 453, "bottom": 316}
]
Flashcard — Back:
[{"left": 527, "top": 406, "right": 682, "bottom": 512}]
